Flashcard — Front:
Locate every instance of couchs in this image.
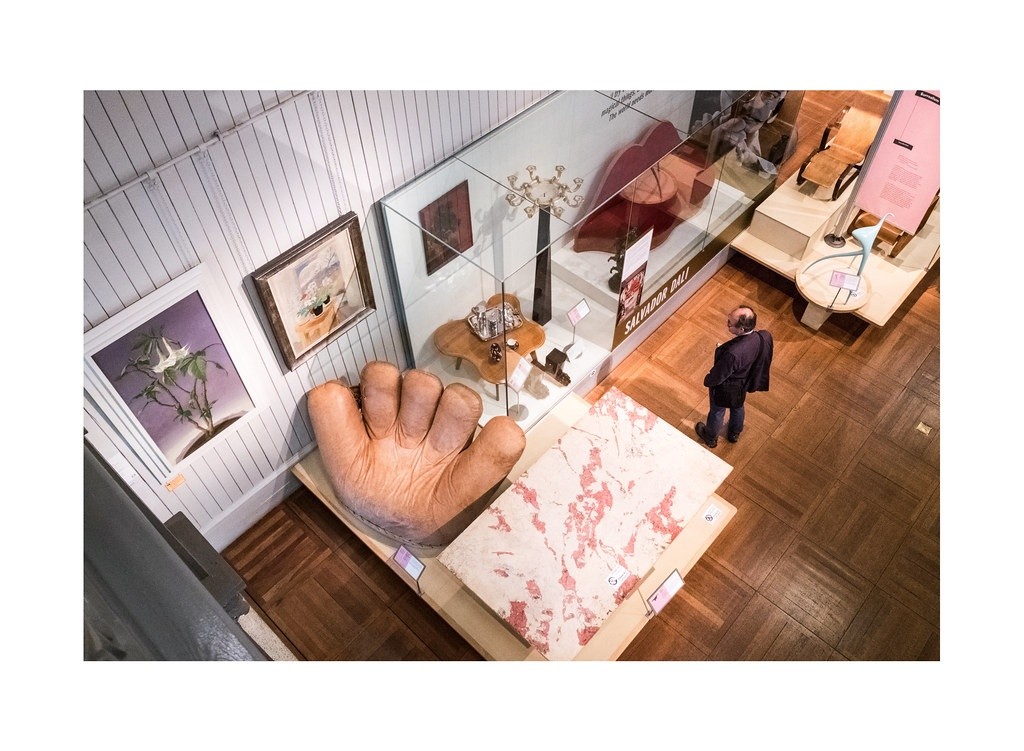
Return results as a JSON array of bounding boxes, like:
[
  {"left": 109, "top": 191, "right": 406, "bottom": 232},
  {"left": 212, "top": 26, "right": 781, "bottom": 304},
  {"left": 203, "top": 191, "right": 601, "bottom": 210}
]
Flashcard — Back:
[{"left": 306, "top": 360, "right": 526, "bottom": 548}]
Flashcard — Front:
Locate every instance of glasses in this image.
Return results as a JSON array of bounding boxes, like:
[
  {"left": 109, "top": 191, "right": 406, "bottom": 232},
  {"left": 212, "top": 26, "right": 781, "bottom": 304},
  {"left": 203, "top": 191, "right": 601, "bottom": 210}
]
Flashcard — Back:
[{"left": 727, "top": 314, "right": 736, "bottom": 327}]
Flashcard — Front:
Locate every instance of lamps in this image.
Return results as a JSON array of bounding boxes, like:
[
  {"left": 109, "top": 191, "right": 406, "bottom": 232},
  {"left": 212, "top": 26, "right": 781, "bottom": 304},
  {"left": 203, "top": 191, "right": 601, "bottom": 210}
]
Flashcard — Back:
[{"left": 506, "top": 163, "right": 584, "bottom": 326}]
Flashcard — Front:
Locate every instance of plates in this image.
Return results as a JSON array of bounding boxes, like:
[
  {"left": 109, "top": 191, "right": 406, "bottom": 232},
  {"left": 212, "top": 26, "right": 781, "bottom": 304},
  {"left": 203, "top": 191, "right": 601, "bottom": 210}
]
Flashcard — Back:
[{"left": 502, "top": 342, "right": 518, "bottom": 352}]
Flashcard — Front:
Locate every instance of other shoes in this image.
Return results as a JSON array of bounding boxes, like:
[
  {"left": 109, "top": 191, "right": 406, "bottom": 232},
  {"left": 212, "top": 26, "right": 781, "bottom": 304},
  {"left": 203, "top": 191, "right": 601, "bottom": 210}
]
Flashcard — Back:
[
  {"left": 696, "top": 421, "right": 717, "bottom": 448},
  {"left": 728, "top": 419, "right": 740, "bottom": 442}
]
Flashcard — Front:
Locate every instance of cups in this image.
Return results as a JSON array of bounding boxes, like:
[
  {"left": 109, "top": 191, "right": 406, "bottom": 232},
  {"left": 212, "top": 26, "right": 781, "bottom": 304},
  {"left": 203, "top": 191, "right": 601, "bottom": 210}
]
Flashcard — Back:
[
  {"left": 477, "top": 305, "right": 514, "bottom": 332},
  {"left": 508, "top": 339, "right": 519, "bottom": 348}
]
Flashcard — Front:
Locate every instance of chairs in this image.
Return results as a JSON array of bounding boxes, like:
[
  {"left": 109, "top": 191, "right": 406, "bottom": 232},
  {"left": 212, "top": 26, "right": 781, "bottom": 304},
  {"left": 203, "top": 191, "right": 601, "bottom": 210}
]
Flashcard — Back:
[{"left": 797, "top": 106, "right": 884, "bottom": 200}]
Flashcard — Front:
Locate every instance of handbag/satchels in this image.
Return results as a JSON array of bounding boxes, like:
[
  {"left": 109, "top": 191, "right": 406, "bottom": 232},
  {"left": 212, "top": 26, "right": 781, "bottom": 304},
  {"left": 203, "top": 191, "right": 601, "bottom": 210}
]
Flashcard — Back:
[{"left": 713, "top": 383, "right": 746, "bottom": 409}]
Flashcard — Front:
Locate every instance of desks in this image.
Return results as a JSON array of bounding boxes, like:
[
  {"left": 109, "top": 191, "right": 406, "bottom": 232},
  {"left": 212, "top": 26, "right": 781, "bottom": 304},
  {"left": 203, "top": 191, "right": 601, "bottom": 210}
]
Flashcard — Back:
[
  {"left": 434, "top": 293, "right": 546, "bottom": 401},
  {"left": 436, "top": 384, "right": 736, "bottom": 661}
]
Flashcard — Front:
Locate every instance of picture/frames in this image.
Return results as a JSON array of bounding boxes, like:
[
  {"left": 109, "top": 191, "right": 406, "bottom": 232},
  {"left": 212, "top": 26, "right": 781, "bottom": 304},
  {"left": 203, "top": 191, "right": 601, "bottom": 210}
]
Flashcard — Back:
[
  {"left": 250, "top": 210, "right": 377, "bottom": 372},
  {"left": 419, "top": 179, "right": 474, "bottom": 276},
  {"left": 84, "top": 261, "right": 267, "bottom": 485}
]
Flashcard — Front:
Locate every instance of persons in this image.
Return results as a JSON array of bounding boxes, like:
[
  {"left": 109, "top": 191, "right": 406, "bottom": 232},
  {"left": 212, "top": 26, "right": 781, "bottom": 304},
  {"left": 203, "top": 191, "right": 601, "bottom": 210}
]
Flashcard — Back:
[
  {"left": 695, "top": 305, "right": 774, "bottom": 448},
  {"left": 690, "top": 90, "right": 789, "bottom": 175}
]
